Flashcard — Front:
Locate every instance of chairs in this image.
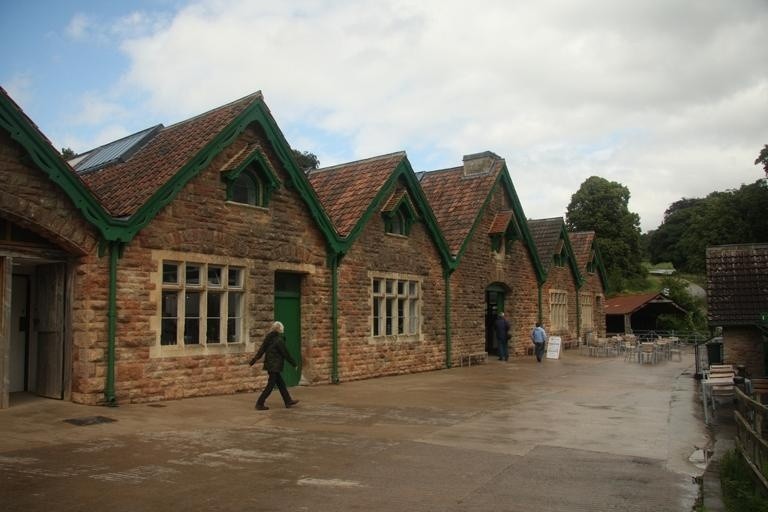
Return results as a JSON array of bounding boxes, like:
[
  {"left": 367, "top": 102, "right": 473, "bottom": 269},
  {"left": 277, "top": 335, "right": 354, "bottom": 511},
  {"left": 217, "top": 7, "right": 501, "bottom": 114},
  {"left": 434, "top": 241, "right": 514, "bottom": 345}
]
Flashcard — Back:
[
  {"left": 578, "top": 333, "right": 682, "bottom": 365},
  {"left": 699, "top": 364, "right": 768, "bottom": 424}
]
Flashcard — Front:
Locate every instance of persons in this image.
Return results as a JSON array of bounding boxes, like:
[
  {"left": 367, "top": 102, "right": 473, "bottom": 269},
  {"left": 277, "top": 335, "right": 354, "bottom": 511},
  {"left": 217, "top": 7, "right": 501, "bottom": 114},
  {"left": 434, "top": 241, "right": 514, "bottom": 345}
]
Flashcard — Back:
[
  {"left": 529, "top": 322, "right": 546, "bottom": 362},
  {"left": 248, "top": 320, "right": 298, "bottom": 410},
  {"left": 494, "top": 312, "right": 509, "bottom": 362}
]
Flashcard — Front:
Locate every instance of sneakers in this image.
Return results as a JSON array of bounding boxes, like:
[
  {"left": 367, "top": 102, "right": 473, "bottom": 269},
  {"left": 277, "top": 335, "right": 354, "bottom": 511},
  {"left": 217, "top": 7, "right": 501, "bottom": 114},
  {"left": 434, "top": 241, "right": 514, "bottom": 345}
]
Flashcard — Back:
[
  {"left": 284, "top": 399, "right": 300, "bottom": 407},
  {"left": 255, "top": 405, "right": 270, "bottom": 410}
]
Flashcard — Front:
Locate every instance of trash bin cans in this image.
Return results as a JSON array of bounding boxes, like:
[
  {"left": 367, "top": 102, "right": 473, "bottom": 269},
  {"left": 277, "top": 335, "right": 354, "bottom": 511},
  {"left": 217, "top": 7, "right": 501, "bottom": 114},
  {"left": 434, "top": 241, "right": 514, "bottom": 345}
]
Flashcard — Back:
[{"left": 705, "top": 342, "right": 723, "bottom": 369}]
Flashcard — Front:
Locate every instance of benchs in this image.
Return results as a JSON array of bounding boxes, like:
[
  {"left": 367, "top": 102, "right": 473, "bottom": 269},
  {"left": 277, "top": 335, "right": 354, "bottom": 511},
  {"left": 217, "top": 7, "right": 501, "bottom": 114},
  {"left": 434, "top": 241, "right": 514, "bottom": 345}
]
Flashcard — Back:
[{"left": 460, "top": 351, "right": 489, "bottom": 367}]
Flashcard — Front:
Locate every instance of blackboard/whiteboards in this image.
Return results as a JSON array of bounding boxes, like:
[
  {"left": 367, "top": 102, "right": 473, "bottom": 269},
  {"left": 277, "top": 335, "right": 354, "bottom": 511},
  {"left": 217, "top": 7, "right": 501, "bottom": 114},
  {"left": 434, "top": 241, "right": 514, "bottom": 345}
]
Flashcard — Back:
[{"left": 546, "top": 336, "right": 561, "bottom": 359}]
